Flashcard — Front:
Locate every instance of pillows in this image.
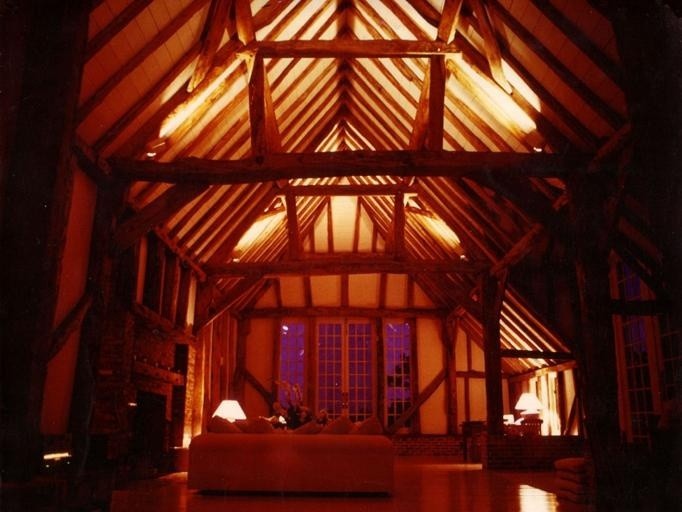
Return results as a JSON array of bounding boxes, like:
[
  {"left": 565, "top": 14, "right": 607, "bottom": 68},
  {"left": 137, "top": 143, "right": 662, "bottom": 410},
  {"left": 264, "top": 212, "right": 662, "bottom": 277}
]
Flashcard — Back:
[{"left": 207, "top": 416, "right": 386, "bottom": 435}]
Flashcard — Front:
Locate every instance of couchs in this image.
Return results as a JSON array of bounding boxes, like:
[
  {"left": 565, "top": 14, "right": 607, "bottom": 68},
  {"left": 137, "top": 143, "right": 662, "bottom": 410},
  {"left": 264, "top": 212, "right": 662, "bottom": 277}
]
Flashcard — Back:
[{"left": 187, "top": 416, "right": 395, "bottom": 496}]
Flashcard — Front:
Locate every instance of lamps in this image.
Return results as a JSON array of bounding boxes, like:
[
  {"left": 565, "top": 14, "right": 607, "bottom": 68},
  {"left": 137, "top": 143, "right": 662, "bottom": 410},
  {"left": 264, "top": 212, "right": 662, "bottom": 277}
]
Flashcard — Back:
[
  {"left": 515, "top": 392, "right": 544, "bottom": 421},
  {"left": 212, "top": 399, "right": 247, "bottom": 422}
]
